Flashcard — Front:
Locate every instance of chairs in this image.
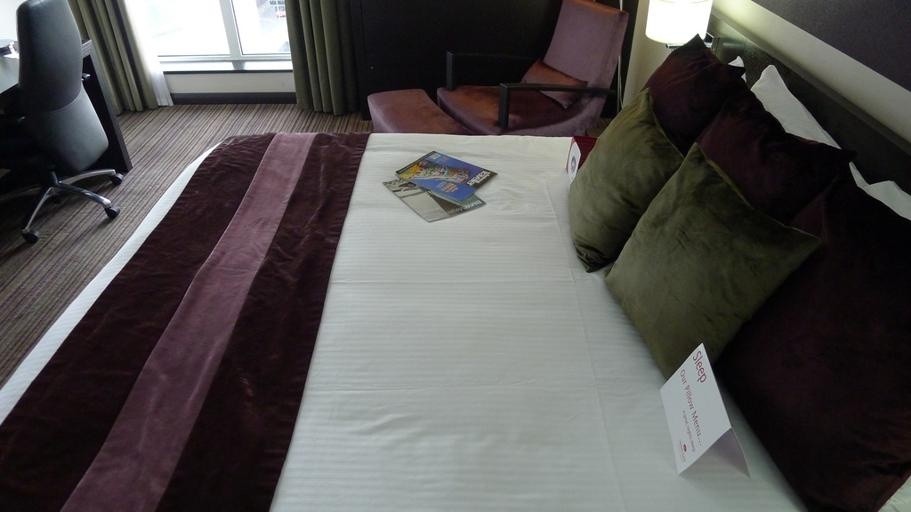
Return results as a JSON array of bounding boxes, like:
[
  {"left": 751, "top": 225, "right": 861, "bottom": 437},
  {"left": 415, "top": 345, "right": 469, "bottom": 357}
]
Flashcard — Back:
[
  {"left": 435, "top": 1, "right": 634, "bottom": 136},
  {"left": 4, "top": 0, "right": 126, "bottom": 243}
]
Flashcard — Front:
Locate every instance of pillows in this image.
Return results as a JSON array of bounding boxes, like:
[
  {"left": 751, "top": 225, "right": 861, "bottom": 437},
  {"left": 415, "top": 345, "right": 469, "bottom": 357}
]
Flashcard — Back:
[{"left": 519, "top": 55, "right": 591, "bottom": 110}]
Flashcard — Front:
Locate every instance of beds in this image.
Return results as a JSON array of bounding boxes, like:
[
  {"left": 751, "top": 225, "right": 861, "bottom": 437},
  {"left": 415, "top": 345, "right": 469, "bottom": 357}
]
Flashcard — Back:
[{"left": 2, "top": 0, "right": 911, "bottom": 512}]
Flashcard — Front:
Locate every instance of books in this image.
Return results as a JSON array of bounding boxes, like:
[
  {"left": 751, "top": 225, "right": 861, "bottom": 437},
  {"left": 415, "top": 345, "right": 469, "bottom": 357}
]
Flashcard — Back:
[
  {"left": 395, "top": 150, "right": 498, "bottom": 206},
  {"left": 381, "top": 179, "right": 487, "bottom": 222}
]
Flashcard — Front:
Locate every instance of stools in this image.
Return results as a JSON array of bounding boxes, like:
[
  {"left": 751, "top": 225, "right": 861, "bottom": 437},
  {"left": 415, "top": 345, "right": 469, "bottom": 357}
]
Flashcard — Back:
[{"left": 366, "top": 86, "right": 470, "bottom": 133}]
